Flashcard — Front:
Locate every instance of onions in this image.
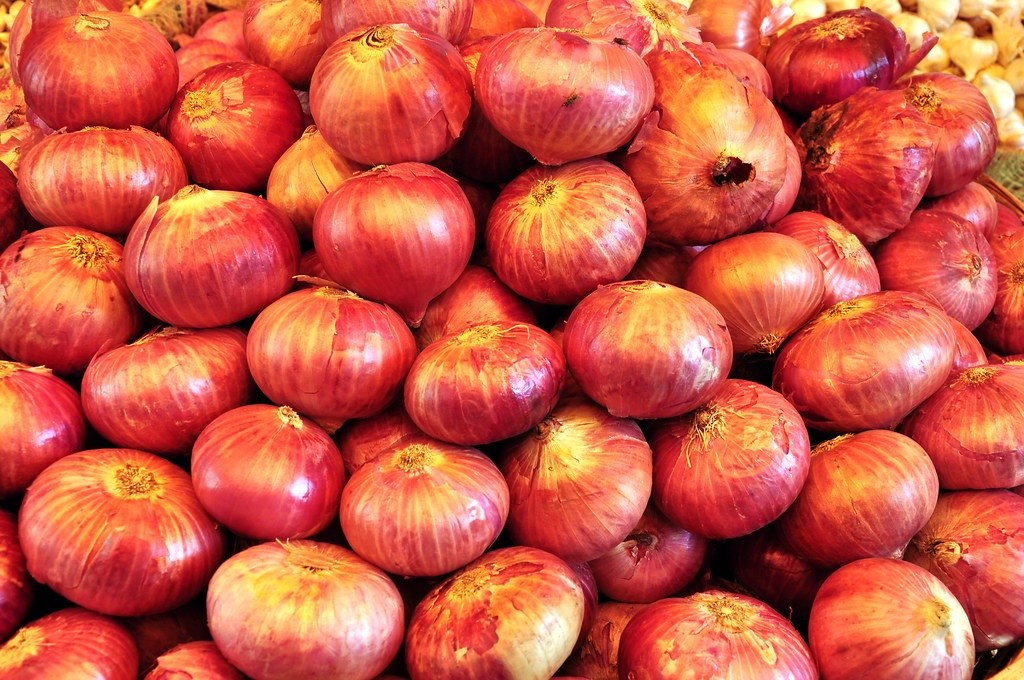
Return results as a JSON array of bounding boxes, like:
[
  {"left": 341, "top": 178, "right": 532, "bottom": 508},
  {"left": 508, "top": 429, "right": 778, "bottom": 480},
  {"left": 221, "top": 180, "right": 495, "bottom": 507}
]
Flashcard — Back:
[{"left": 0, "top": 0, "right": 1024, "bottom": 680}]
